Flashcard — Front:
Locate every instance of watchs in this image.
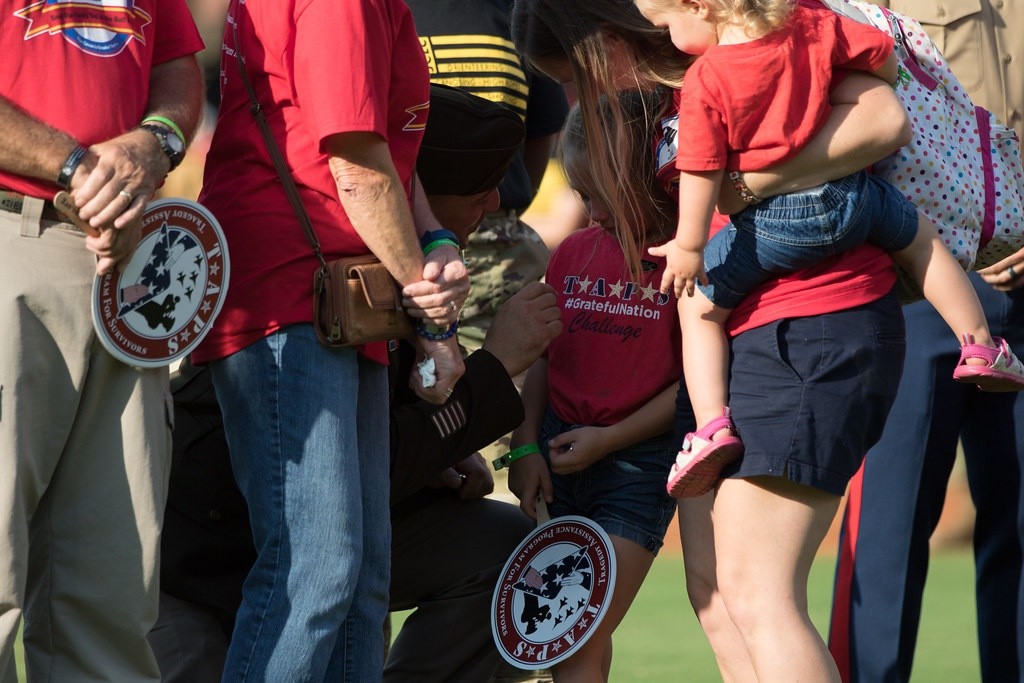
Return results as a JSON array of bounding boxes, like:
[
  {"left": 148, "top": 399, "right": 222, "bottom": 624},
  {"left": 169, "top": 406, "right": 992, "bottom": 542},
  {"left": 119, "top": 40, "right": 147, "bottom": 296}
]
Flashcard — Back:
[{"left": 141, "top": 124, "right": 184, "bottom": 167}]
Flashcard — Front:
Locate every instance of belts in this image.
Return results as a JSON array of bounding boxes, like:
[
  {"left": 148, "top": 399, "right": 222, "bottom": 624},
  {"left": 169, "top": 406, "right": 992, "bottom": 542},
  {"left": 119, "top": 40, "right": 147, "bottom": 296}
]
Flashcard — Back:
[{"left": 0, "top": 189, "right": 74, "bottom": 224}]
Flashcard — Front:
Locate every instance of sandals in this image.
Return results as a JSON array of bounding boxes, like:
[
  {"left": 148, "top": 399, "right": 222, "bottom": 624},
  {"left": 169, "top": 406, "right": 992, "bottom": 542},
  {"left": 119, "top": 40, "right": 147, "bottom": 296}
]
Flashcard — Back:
[
  {"left": 667, "top": 404, "right": 744, "bottom": 498},
  {"left": 953, "top": 330, "right": 1024, "bottom": 391}
]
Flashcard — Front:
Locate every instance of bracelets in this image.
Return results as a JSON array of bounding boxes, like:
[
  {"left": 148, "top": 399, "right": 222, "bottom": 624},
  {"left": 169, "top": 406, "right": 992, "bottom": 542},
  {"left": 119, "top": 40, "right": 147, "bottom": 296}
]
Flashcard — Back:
[
  {"left": 491, "top": 442, "right": 542, "bottom": 469},
  {"left": 55, "top": 143, "right": 90, "bottom": 186},
  {"left": 727, "top": 170, "right": 761, "bottom": 206},
  {"left": 416, "top": 316, "right": 458, "bottom": 341},
  {"left": 141, "top": 115, "right": 188, "bottom": 149},
  {"left": 421, "top": 229, "right": 462, "bottom": 256}
]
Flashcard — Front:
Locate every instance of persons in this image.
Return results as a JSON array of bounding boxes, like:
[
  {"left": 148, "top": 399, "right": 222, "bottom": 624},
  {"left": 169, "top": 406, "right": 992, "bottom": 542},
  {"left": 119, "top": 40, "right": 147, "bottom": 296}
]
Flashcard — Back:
[
  {"left": 406, "top": 0, "right": 572, "bottom": 224},
  {"left": 541, "top": 0, "right": 902, "bottom": 683},
  {"left": 162, "top": 83, "right": 564, "bottom": 603},
  {"left": 0, "top": 0, "right": 206, "bottom": 682},
  {"left": 626, "top": 0, "right": 1023, "bottom": 500},
  {"left": 495, "top": 93, "right": 691, "bottom": 683},
  {"left": 193, "top": 2, "right": 475, "bottom": 683},
  {"left": 829, "top": 0, "right": 1024, "bottom": 683}
]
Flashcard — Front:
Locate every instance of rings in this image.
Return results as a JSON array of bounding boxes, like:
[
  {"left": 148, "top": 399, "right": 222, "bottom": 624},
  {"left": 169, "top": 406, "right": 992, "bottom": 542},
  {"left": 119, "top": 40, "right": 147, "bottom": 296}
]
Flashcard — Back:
[
  {"left": 120, "top": 191, "right": 133, "bottom": 201},
  {"left": 445, "top": 388, "right": 452, "bottom": 397},
  {"left": 1008, "top": 265, "right": 1015, "bottom": 277},
  {"left": 449, "top": 300, "right": 457, "bottom": 311}
]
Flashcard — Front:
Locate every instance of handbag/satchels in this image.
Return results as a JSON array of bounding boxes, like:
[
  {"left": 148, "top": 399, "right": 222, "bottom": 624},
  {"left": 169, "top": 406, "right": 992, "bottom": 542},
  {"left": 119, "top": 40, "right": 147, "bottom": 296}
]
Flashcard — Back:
[{"left": 315, "top": 254, "right": 415, "bottom": 344}]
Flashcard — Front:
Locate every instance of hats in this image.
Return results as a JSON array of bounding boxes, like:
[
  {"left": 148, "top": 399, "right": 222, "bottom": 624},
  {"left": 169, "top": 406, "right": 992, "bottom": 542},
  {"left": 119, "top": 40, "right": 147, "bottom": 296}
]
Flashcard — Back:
[{"left": 416, "top": 81, "right": 526, "bottom": 196}]
